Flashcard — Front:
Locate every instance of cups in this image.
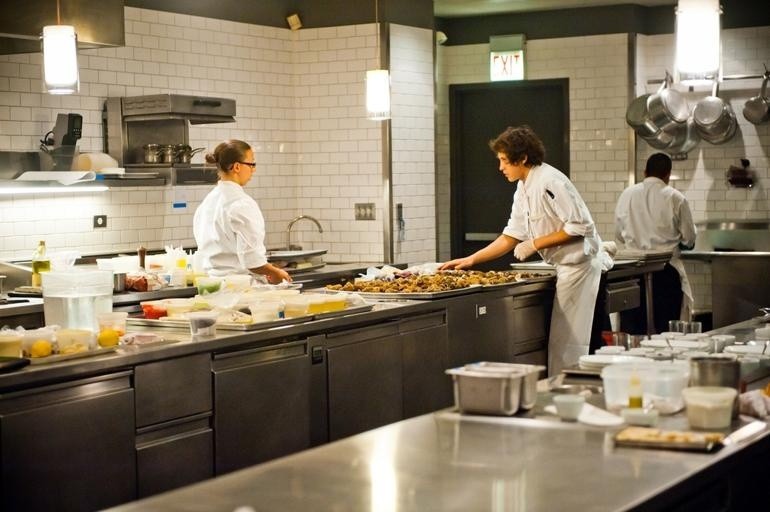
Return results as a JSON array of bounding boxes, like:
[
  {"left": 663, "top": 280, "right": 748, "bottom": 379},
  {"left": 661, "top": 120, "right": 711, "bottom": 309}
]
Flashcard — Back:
[
  {"left": 1, "top": 311, "right": 128, "bottom": 360},
  {"left": 188, "top": 277, "right": 347, "bottom": 337},
  {"left": 681, "top": 384, "right": 738, "bottom": 431}
]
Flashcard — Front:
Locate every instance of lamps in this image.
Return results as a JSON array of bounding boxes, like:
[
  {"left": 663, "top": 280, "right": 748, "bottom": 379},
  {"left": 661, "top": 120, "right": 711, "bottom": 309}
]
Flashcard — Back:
[
  {"left": 39, "top": 0, "right": 80, "bottom": 95},
  {"left": 365, "top": 0, "right": 391, "bottom": 121},
  {"left": 672, "top": 0, "right": 724, "bottom": 87},
  {"left": 0, "top": 186, "right": 112, "bottom": 194}
]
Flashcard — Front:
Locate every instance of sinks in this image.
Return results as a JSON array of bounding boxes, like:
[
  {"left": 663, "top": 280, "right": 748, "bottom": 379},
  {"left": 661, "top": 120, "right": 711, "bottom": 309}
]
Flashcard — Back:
[{"left": 322, "top": 260, "right": 349, "bottom": 266}]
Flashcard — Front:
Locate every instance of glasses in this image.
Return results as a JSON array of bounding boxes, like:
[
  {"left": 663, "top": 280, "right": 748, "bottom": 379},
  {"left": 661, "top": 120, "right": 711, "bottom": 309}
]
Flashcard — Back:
[{"left": 230, "top": 161, "right": 256, "bottom": 170}]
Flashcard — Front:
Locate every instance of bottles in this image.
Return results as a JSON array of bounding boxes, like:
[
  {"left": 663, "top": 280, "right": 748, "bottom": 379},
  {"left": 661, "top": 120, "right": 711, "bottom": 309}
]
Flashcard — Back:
[
  {"left": 31, "top": 240, "right": 52, "bottom": 289},
  {"left": 137, "top": 244, "right": 146, "bottom": 272}
]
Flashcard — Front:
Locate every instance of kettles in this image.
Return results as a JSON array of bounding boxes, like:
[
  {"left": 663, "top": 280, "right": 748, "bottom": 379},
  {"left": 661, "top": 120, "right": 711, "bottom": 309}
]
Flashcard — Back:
[{"left": 142, "top": 143, "right": 205, "bottom": 165}]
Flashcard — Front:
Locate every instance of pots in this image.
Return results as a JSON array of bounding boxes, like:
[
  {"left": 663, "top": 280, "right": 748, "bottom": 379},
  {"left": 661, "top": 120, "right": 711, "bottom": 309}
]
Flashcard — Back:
[{"left": 625, "top": 70, "right": 770, "bottom": 156}]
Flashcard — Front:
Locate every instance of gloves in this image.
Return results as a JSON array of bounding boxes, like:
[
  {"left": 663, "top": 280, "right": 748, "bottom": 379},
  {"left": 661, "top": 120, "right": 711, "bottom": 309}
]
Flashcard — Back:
[{"left": 514, "top": 239, "right": 538, "bottom": 261}]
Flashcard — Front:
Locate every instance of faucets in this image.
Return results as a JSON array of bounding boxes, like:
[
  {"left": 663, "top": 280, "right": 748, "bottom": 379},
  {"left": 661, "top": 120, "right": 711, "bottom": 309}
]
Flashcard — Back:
[{"left": 286, "top": 215, "right": 322, "bottom": 251}]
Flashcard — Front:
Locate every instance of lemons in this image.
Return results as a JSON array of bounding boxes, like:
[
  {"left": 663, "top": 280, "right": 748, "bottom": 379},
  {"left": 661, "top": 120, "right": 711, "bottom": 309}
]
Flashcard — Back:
[
  {"left": 98, "top": 328, "right": 120, "bottom": 348},
  {"left": 31, "top": 340, "right": 52, "bottom": 358}
]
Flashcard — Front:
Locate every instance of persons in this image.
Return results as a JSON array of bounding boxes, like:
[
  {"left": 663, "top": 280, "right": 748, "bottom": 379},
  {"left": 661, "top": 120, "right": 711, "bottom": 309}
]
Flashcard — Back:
[
  {"left": 438, "top": 126, "right": 614, "bottom": 377},
  {"left": 609, "top": 153, "right": 697, "bottom": 333},
  {"left": 192, "top": 140, "right": 293, "bottom": 286}
]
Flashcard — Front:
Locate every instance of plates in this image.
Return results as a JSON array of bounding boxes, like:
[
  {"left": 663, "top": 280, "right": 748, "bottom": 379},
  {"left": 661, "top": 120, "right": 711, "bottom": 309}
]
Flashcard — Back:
[{"left": 577, "top": 330, "right": 770, "bottom": 382}]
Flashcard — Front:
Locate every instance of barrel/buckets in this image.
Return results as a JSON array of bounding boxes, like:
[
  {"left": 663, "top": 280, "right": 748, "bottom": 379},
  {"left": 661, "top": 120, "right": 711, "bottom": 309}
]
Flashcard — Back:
[{"left": 38, "top": 268, "right": 113, "bottom": 337}]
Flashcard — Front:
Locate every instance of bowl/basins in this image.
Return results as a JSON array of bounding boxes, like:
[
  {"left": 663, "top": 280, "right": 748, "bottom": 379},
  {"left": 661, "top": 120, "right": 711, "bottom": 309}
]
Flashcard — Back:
[
  {"left": 113, "top": 271, "right": 126, "bottom": 293},
  {"left": 551, "top": 393, "right": 588, "bottom": 422}
]
